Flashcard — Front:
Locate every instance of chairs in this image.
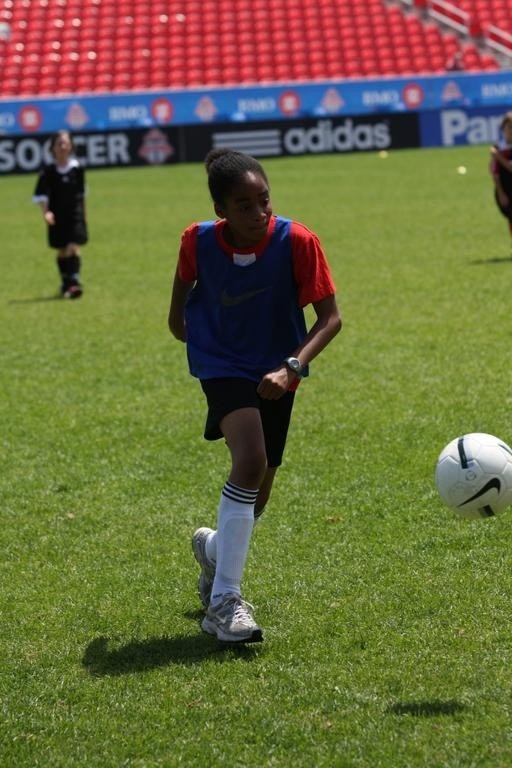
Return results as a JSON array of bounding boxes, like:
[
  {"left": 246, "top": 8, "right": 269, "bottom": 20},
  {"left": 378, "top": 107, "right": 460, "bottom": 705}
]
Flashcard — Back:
[{"left": 0, "top": 0, "right": 511, "bottom": 105}]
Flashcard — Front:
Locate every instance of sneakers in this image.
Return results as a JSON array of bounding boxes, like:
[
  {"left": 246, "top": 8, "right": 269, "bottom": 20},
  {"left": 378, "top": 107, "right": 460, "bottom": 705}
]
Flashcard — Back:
[
  {"left": 192, "top": 527, "right": 215, "bottom": 607},
  {"left": 202, "top": 595, "right": 263, "bottom": 642}
]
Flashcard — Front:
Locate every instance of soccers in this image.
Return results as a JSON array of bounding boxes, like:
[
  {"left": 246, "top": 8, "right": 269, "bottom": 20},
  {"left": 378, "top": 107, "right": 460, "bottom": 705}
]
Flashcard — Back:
[{"left": 435, "top": 433, "right": 512, "bottom": 519}]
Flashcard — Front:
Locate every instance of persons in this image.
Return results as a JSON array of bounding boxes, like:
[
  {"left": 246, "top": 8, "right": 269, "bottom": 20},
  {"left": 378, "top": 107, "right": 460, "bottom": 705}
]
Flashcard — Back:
[
  {"left": 31, "top": 130, "right": 89, "bottom": 297},
  {"left": 490, "top": 110, "right": 511, "bottom": 234},
  {"left": 167, "top": 147, "right": 343, "bottom": 640}
]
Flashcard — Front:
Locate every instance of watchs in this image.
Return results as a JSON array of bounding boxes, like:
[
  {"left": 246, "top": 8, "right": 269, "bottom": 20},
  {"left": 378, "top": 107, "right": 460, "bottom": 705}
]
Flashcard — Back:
[{"left": 279, "top": 356, "right": 304, "bottom": 377}]
